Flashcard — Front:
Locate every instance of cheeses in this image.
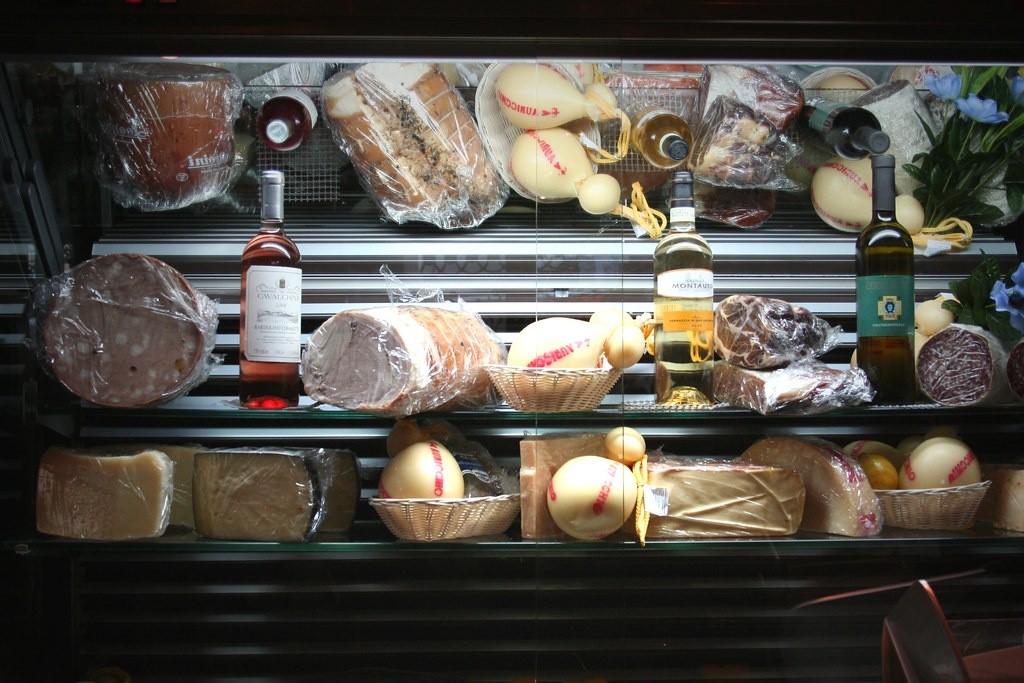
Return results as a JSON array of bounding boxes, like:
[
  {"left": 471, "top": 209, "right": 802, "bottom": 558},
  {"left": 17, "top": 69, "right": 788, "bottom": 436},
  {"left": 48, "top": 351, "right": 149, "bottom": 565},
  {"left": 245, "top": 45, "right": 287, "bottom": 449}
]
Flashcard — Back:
[{"left": 33, "top": 441, "right": 208, "bottom": 537}]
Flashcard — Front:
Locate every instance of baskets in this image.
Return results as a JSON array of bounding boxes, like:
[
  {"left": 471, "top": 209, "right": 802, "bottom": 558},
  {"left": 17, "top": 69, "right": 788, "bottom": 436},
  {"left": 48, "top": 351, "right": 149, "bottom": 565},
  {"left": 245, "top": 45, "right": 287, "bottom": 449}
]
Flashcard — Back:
[
  {"left": 369, "top": 493, "right": 521, "bottom": 540},
  {"left": 872, "top": 480, "right": 991, "bottom": 531},
  {"left": 483, "top": 364, "right": 624, "bottom": 413}
]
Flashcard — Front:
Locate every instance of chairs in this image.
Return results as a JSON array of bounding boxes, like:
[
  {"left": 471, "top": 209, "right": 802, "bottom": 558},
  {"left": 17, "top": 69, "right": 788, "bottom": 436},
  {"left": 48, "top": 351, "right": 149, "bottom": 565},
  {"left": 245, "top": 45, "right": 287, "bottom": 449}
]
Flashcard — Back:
[{"left": 879, "top": 579, "right": 1024, "bottom": 683}]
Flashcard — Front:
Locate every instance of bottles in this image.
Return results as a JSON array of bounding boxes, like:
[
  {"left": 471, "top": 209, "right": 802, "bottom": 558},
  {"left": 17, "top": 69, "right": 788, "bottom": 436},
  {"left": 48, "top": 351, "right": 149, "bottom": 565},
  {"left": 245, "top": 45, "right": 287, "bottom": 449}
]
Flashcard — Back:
[
  {"left": 795, "top": 96, "right": 891, "bottom": 162},
  {"left": 256, "top": 89, "right": 318, "bottom": 151},
  {"left": 855, "top": 155, "right": 917, "bottom": 405},
  {"left": 652, "top": 170, "right": 715, "bottom": 406},
  {"left": 630, "top": 105, "right": 693, "bottom": 168},
  {"left": 239, "top": 170, "right": 303, "bottom": 409}
]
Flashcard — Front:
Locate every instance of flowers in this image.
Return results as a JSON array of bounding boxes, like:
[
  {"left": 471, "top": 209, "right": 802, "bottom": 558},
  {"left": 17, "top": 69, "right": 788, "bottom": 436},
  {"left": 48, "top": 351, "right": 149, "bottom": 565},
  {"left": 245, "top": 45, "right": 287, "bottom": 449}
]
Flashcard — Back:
[
  {"left": 903, "top": 67, "right": 1024, "bottom": 246},
  {"left": 945, "top": 253, "right": 1023, "bottom": 344}
]
